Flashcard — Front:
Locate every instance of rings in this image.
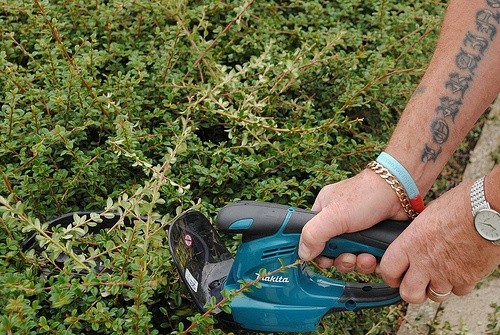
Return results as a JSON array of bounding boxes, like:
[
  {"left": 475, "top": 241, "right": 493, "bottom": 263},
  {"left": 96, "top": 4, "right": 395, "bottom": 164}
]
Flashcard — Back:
[{"left": 429, "top": 289, "right": 451, "bottom": 299}]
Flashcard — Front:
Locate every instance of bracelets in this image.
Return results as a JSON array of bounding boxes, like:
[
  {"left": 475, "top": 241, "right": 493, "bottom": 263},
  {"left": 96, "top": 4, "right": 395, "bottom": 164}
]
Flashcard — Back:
[
  {"left": 365, "top": 161, "right": 419, "bottom": 221},
  {"left": 376, "top": 152, "right": 425, "bottom": 214}
]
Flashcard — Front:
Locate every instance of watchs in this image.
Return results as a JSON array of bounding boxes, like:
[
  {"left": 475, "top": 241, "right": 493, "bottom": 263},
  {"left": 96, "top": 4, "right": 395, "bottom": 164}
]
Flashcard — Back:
[{"left": 470, "top": 175, "right": 500, "bottom": 247}]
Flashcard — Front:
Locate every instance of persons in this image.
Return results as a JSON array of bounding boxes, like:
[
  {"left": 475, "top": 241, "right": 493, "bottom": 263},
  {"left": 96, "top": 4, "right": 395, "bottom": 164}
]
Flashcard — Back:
[{"left": 297, "top": 0, "right": 500, "bottom": 305}]
